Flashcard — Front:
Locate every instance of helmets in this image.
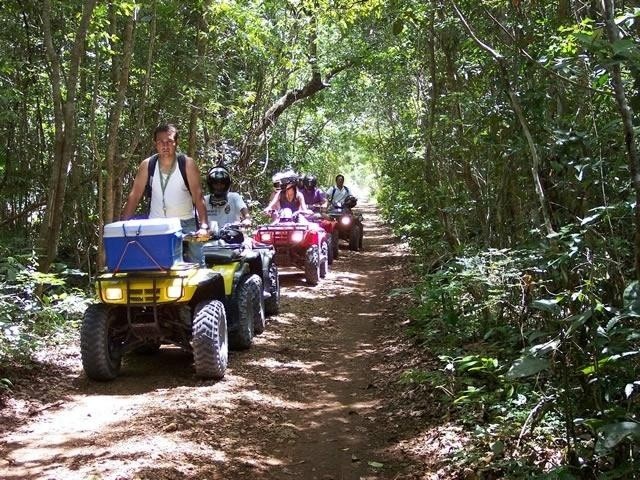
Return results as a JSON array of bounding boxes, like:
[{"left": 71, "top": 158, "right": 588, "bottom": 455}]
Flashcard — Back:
[
  {"left": 271, "top": 169, "right": 319, "bottom": 192},
  {"left": 207, "top": 166, "right": 231, "bottom": 195}
]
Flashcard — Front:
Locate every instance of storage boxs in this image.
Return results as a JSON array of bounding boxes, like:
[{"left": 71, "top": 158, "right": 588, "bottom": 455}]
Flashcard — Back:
[{"left": 105, "top": 217, "right": 185, "bottom": 272}]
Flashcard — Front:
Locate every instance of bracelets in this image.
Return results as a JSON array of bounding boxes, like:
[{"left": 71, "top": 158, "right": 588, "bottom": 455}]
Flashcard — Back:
[{"left": 200, "top": 224, "right": 208, "bottom": 229}]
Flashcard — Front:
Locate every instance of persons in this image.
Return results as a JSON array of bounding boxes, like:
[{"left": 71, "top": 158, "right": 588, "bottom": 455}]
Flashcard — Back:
[
  {"left": 326, "top": 175, "right": 352, "bottom": 213},
  {"left": 196, "top": 167, "right": 251, "bottom": 245},
  {"left": 261, "top": 171, "right": 325, "bottom": 225},
  {"left": 121, "top": 125, "right": 208, "bottom": 268}
]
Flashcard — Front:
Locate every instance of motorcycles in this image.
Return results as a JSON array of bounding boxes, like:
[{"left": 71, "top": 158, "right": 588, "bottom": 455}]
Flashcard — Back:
[
  {"left": 80, "top": 229, "right": 254, "bottom": 383},
  {"left": 246, "top": 200, "right": 366, "bottom": 286},
  {"left": 196, "top": 224, "right": 281, "bottom": 335}
]
